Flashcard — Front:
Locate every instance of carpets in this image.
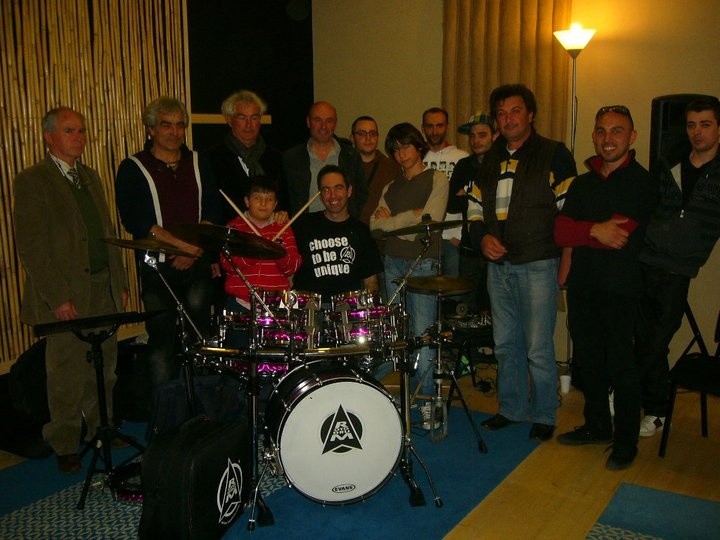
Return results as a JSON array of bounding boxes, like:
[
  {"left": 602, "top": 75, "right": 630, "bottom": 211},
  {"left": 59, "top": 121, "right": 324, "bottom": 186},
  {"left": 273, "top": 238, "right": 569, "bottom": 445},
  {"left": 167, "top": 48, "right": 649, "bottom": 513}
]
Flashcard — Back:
[
  {"left": 583, "top": 481, "right": 720, "bottom": 539},
  {"left": 1, "top": 393, "right": 557, "bottom": 539}
]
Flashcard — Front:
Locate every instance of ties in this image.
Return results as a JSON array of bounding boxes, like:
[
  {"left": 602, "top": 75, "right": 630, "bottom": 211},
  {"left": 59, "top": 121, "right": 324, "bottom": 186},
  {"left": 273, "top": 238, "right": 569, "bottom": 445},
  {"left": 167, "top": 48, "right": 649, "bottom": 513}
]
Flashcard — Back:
[{"left": 68, "top": 168, "right": 81, "bottom": 188}]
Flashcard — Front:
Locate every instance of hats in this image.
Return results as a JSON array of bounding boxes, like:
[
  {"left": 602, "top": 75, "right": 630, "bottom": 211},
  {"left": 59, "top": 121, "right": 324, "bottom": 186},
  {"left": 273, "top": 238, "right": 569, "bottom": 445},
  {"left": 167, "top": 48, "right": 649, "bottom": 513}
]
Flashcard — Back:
[{"left": 458, "top": 111, "right": 499, "bottom": 136}]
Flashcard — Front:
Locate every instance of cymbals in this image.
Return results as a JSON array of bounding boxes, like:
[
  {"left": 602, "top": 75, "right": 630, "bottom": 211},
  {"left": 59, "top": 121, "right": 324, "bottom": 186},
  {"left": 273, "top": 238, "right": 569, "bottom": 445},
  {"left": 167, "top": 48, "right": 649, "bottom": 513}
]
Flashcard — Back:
[
  {"left": 383, "top": 219, "right": 472, "bottom": 236},
  {"left": 395, "top": 274, "right": 472, "bottom": 294},
  {"left": 172, "top": 222, "right": 287, "bottom": 260},
  {"left": 97, "top": 236, "right": 198, "bottom": 259}
]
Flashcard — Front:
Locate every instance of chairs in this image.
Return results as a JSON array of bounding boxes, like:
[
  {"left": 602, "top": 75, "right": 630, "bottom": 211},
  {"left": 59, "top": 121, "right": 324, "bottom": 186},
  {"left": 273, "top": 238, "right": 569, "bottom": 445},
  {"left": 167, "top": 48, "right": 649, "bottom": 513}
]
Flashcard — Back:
[{"left": 658, "top": 295, "right": 720, "bottom": 460}]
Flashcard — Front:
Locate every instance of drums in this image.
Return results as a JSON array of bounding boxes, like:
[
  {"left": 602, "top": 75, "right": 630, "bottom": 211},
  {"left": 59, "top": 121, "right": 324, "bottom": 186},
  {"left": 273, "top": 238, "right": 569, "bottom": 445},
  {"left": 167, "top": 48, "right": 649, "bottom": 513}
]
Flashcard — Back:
[
  {"left": 265, "top": 358, "right": 405, "bottom": 505},
  {"left": 214, "top": 284, "right": 399, "bottom": 375}
]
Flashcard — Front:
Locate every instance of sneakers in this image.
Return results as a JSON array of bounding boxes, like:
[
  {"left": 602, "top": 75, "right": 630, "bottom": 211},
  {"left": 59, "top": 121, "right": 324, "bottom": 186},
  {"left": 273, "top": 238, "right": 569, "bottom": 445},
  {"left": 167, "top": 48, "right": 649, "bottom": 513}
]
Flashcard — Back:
[
  {"left": 421, "top": 404, "right": 440, "bottom": 429},
  {"left": 108, "top": 436, "right": 131, "bottom": 447},
  {"left": 637, "top": 415, "right": 671, "bottom": 436},
  {"left": 58, "top": 453, "right": 82, "bottom": 473},
  {"left": 483, "top": 414, "right": 528, "bottom": 430},
  {"left": 559, "top": 423, "right": 611, "bottom": 445},
  {"left": 604, "top": 442, "right": 638, "bottom": 470},
  {"left": 531, "top": 423, "right": 554, "bottom": 440}
]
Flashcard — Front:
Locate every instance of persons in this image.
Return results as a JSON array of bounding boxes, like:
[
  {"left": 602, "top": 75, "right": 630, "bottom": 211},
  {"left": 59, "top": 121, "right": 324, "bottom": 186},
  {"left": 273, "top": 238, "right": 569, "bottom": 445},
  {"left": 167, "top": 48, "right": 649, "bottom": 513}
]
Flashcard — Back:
[
  {"left": 556, "top": 105, "right": 660, "bottom": 471},
  {"left": 370, "top": 122, "right": 448, "bottom": 434},
  {"left": 401, "top": 107, "right": 468, "bottom": 279},
  {"left": 447, "top": 111, "right": 515, "bottom": 377},
  {"left": 114, "top": 97, "right": 227, "bottom": 445},
  {"left": 273, "top": 162, "right": 394, "bottom": 384},
  {"left": 205, "top": 90, "right": 284, "bottom": 314},
  {"left": 9, "top": 106, "right": 130, "bottom": 472},
  {"left": 476, "top": 82, "right": 579, "bottom": 443},
  {"left": 283, "top": 100, "right": 370, "bottom": 220},
  {"left": 609, "top": 94, "right": 719, "bottom": 432},
  {"left": 219, "top": 175, "right": 302, "bottom": 315},
  {"left": 352, "top": 115, "right": 401, "bottom": 301}
]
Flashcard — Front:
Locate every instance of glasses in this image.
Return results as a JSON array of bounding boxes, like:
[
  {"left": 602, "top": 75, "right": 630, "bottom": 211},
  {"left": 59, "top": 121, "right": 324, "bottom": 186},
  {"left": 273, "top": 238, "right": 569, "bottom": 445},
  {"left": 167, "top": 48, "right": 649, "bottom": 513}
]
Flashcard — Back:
[
  {"left": 352, "top": 130, "right": 378, "bottom": 137},
  {"left": 595, "top": 104, "right": 635, "bottom": 128}
]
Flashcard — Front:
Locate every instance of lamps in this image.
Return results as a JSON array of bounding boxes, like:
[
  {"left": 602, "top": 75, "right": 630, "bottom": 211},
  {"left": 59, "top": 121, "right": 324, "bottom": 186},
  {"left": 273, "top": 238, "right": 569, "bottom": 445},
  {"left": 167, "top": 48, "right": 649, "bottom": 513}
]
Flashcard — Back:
[{"left": 548, "top": 22, "right": 598, "bottom": 157}]
